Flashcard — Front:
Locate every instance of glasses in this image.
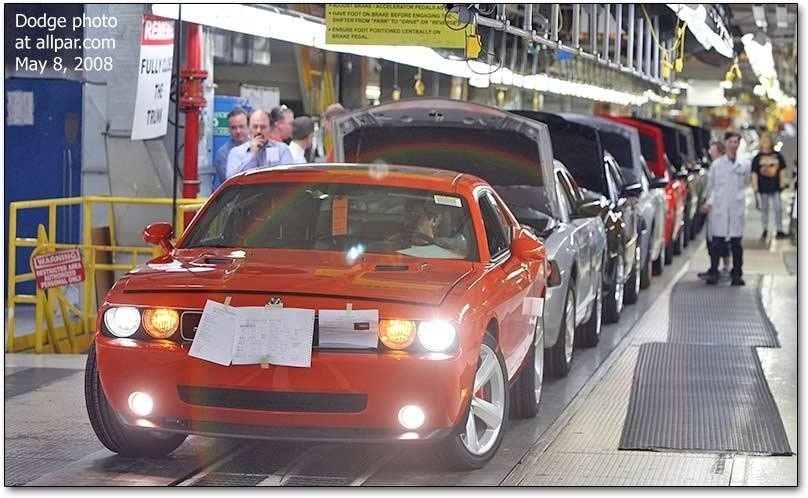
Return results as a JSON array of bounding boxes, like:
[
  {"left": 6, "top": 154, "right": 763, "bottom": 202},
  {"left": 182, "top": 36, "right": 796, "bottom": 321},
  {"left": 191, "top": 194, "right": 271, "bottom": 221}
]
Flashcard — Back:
[{"left": 274, "top": 103, "right": 289, "bottom": 118}]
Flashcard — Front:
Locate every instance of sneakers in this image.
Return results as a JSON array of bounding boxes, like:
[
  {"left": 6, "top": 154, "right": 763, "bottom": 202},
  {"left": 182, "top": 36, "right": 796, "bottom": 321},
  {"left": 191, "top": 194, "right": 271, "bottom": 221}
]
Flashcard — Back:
[
  {"left": 697, "top": 269, "right": 745, "bottom": 286},
  {"left": 761, "top": 229, "right": 789, "bottom": 238}
]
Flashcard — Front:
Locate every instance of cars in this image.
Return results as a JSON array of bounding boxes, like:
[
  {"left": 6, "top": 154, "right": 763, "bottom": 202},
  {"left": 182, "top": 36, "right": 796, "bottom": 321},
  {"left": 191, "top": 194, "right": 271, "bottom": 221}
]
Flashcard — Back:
[{"left": 83, "top": 158, "right": 553, "bottom": 474}]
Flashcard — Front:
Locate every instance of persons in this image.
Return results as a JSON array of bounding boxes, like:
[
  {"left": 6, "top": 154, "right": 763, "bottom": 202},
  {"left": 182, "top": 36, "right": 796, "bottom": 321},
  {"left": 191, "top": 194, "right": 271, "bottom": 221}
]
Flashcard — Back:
[
  {"left": 706, "top": 131, "right": 753, "bottom": 286},
  {"left": 696, "top": 142, "right": 731, "bottom": 282},
  {"left": 750, "top": 132, "right": 790, "bottom": 240},
  {"left": 214, "top": 101, "right": 352, "bottom": 186},
  {"left": 381, "top": 199, "right": 468, "bottom": 259}
]
